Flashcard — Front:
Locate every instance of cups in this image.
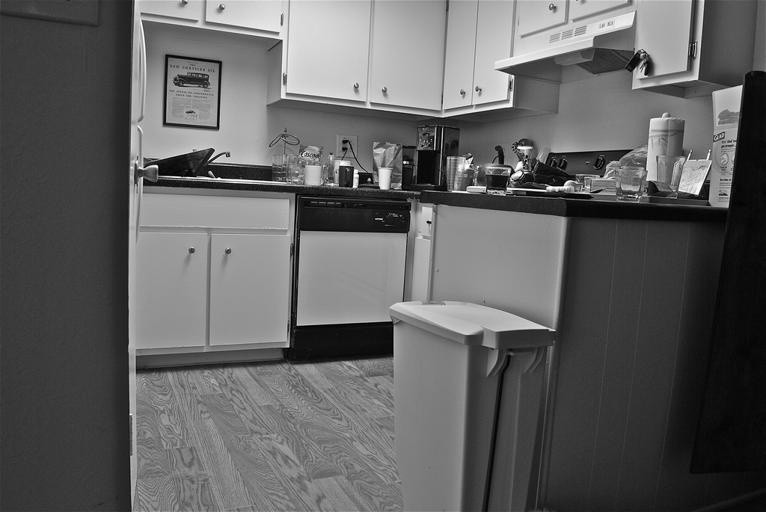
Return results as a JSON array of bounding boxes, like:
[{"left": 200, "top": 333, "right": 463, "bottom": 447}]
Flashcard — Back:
[
  {"left": 446, "top": 155, "right": 479, "bottom": 191},
  {"left": 271, "top": 152, "right": 359, "bottom": 188},
  {"left": 569, "top": 154, "right": 683, "bottom": 203},
  {"left": 378, "top": 167, "right": 393, "bottom": 190}
]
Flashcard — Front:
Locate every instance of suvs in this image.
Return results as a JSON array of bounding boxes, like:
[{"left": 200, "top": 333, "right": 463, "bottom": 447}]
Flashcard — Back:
[{"left": 173, "top": 71, "right": 211, "bottom": 90}]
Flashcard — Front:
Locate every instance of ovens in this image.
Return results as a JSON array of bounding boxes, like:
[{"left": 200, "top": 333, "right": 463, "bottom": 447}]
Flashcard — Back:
[{"left": 290, "top": 194, "right": 411, "bottom": 363}]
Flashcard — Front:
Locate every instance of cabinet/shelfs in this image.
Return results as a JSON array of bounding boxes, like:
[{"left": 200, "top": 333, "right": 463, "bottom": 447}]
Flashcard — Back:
[
  {"left": 133, "top": 226, "right": 290, "bottom": 353},
  {"left": 634, "top": 0, "right": 756, "bottom": 98},
  {"left": 140, "top": 1, "right": 282, "bottom": 53},
  {"left": 266, "top": 2, "right": 446, "bottom": 117},
  {"left": 516, "top": 1, "right": 631, "bottom": 38},
  {"left": 444, "top": 1, "right": 559, "bottom": 121}
]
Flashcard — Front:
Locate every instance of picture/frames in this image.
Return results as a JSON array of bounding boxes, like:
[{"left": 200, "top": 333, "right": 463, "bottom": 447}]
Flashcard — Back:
[{"left": 162, "top": 52, "right": 223, "bottom": 130}]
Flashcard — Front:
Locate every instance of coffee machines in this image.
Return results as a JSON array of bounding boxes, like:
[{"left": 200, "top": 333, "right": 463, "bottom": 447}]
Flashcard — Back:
[{"left": 402, "top": 125, "right": 459, "bottom": 190}]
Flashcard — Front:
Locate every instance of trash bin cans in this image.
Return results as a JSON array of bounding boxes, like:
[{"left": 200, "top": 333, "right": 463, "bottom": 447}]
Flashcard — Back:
[{"left": 389, "top": 300, "right": 558, "bottom": 512}]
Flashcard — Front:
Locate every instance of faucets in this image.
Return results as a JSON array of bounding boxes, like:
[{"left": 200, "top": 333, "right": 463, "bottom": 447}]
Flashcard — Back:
[{"left": 202, "top": 151, "right": 230, "bottom": 177}]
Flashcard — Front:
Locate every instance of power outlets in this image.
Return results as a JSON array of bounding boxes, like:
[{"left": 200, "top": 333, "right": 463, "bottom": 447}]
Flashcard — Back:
[{"left": 336, "top": 133, "right": 357, "bottom": 157}]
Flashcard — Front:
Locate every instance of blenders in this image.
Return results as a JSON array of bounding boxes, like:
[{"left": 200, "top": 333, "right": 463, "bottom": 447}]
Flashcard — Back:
[
  {"left": 484, "top": 138, "right": 533, "bottom": 196},
  {"left": 401, "top": 145, "right": 417, "bottom": 190}
]
